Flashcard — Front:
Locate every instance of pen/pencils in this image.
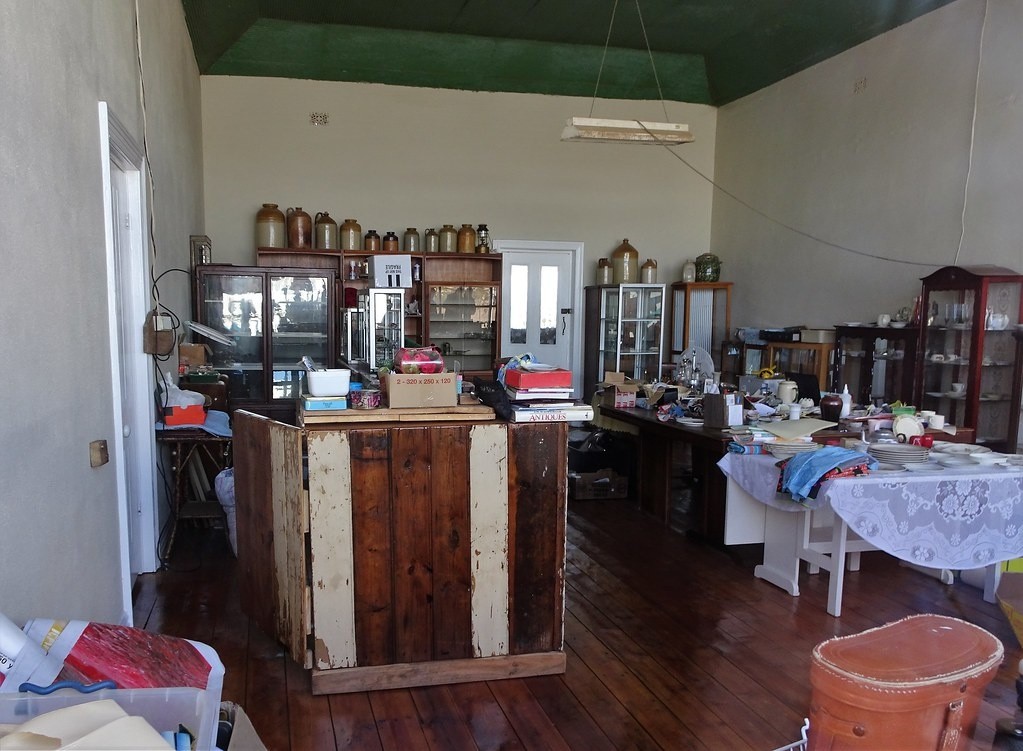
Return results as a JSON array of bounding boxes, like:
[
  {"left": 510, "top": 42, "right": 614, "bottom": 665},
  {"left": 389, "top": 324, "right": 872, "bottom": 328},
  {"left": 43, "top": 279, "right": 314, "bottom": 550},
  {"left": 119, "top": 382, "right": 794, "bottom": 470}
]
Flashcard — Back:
[
  {"left": 719, "top": 385, "right": 728, "bottom": 395},
  {"left": 736, "top": 396, "right": 741, "bottom": 404}
]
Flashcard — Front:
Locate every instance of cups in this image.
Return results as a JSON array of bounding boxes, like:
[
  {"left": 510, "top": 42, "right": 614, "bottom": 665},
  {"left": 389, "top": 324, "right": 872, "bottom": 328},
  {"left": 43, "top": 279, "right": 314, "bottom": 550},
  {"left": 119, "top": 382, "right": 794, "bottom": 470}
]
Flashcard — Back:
[
  {"left": 868, "top": 418, "right": 892, "bottom": 434},
  {"left": 928, "top": 415, "right": 944, "bottom": 430},
  {"left": 789, "top": 404, "right": 800, "bottom": 421},
  {"left": 877, "top": 314, "right": 890, "bottom": 328},
  {"left": 915, "top": 410, "right": 936, "bottom": 421},
  {"left": 443, "top": 343, "right": 450, "bottom": 356},
  {"left": 746, "top": 413, "right": 783, "bottom": 427},
  {"left": 929, "top": 301, "right": 1010, "bottom": 392}
]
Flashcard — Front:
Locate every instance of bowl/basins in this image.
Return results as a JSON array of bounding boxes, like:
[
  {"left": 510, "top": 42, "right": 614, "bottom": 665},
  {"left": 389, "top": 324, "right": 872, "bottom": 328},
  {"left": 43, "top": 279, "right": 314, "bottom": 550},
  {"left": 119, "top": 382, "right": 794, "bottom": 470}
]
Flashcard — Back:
[{"left": 842, "top": 348, "right": 904, "bottom": 359}]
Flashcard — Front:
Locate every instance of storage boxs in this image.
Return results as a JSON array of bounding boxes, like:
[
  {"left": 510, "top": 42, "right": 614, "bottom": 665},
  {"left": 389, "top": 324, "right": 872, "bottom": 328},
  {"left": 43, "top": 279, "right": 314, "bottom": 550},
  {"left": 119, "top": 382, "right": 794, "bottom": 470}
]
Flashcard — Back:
[
  {"left": 893, "top": 406, "right": 916, "bottom": 415},
  {"left": 596, "top": 372, "right": 639, "bottom": 409},
  {"left": 376, "top": 367, "right": 457, "bottom": 409},
  {"left": 840, "top": 436, "right": 859, "bottom": 449},
  {"left": 0, "top": 679, "right": 268, "bottom": 751},
  {"left": 157, "top": 342, "right": 213, "bottom": 424},
  {"left": 300, "top": 393, "right": 347, "bottom": 410},
  {"left": 569, "top": 468, "right": 627, "bottom": 500},
  {"left": 506, "top": 368, "right": 572, "bottom": 390},
  {"left": 703, "top": 393, "right": 745, "bottom": 429},
  {"left": 735, "top": 324, "right": 836, "bottom": 344},
  {"left": 736, "top": 373, "right": 785, "bottom": 396}
]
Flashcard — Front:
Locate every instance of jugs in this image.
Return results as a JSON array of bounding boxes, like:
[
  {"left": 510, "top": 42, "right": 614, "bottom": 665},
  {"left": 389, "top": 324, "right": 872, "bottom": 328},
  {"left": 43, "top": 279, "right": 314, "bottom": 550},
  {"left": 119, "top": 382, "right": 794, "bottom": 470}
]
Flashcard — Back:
[{"left": 776, "top": 381, "right": 797, "bottom": 404}]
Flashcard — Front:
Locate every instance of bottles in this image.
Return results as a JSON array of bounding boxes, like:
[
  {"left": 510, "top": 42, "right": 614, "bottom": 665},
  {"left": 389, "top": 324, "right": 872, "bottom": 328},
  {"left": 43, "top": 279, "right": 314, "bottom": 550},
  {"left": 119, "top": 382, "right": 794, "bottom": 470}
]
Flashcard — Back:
[
  {"left": 594, "top": 237, "right": 719, "bottom": 284},
  {"left": 255, "top": 203, "right": 478, "bottom": 255},
  {"left": 676, "top": 350, "right": 701, "bottom": 390},
  {"left": 840, "top": 384, "right": 851, "bottom": 418},
  {"left": 819, "top": 394, "right": 842, "bottom": 423}
]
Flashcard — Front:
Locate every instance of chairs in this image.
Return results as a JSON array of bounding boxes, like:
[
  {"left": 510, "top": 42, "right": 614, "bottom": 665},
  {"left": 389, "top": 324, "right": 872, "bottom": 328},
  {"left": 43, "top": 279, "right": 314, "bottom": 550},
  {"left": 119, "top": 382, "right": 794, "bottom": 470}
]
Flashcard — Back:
[{"left": 785, "top": 371, "right": 821, "bottom": 407}]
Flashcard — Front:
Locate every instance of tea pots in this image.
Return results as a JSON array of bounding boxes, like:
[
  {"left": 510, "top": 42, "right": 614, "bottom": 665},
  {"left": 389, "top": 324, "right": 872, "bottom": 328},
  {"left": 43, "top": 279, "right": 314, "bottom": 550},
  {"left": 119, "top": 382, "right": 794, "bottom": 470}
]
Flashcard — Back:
[{"left": 860, "top": 427, "right": 907, "bottom": 444}]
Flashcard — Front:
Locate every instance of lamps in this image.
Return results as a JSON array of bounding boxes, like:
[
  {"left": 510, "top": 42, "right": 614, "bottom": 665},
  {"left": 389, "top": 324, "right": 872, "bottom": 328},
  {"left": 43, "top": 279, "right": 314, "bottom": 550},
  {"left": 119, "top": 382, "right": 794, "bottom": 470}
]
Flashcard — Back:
[{"left": 561, "top": 0, "right": 696, "bottom": 146}]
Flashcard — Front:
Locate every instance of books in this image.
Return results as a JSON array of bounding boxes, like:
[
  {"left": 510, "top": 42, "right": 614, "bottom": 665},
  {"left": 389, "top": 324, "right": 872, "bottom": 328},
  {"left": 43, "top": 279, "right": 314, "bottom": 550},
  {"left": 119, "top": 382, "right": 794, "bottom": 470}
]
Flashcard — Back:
[{"left": 505, "top": 386, "right": 594, "bottom": 423}]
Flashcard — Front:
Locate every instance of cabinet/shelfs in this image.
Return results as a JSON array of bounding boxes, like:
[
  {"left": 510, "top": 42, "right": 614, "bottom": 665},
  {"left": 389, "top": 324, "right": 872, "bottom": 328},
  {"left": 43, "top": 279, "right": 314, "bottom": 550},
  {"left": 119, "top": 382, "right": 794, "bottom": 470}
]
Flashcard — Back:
[
  {"left": 833, "top": 264, "right": 1023, "bottom": 454},
  {"left": 721, "top": 341, "right": 834, "bottom": 391},
  {"left": 154, "top": 375, "right": 235, "bottom": 571},
  {"left": 232, "top": 399, "right": 567, "bottom": 694},
  {"left": 584, "top": 284, "right": 666, "bottom": 403},
  {"left": 190, "top": 234, "right": 503, "bottom": 424},
  {"left": 597, "top": 404, "right": 974, "bottom": 546}
]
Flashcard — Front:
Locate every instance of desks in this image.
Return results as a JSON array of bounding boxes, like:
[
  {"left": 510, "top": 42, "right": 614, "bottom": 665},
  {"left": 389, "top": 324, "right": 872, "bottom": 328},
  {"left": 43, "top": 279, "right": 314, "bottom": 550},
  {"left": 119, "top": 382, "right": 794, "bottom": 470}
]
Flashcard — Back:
[{"left": 716, "top": 452, "right": 1023, "bottom": 617}]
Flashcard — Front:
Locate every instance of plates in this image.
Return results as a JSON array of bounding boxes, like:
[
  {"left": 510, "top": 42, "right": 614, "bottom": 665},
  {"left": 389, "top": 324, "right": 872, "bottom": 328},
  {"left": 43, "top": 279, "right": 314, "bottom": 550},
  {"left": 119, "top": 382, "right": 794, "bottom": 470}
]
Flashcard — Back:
[
  {"left": 523, "top": 364, "right": 560, "bottom": 372},
  {"left": 760, "top": 439, "right": 818, "bottom": 460},
  {"left": 867, "top": 441, "right": 1023, "bottom": 475},
  {"left": 893, "top": 414, "right": 925, "bottom": 443},
  {"left": 676, "top": 418, "right": 704, "bottom": 426},
  {"left": 843, "top": 322, "right": 908, "bottom": 328},
  {"left": 850, "top": 422, "right": 863, "bottom": 428},
  {"left": 945, "top": 323, "right": 1006, "bottom": 442}
]
